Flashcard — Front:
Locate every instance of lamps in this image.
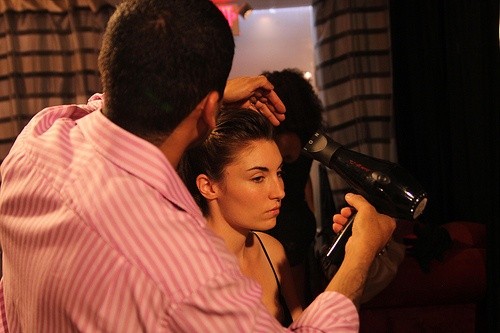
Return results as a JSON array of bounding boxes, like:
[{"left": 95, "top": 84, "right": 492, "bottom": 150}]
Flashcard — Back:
[{"left": 238, "top": 3, "right": 253, "bottom": 21}]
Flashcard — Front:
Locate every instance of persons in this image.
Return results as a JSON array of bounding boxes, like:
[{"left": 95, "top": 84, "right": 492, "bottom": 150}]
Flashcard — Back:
[
  {"left": 0, "top": 0, "right": 397, "bottom": 333},
  {"left": 259, "top": 68, "right": 326, "bottom": 327},
  {"left": 176, "top": 108, "right": 303, "bottom": 327}
]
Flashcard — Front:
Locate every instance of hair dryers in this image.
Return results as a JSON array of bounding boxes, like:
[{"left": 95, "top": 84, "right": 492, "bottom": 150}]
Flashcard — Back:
[{"left": 300, "top": 129, "right": 429, "bottom": 257}]
